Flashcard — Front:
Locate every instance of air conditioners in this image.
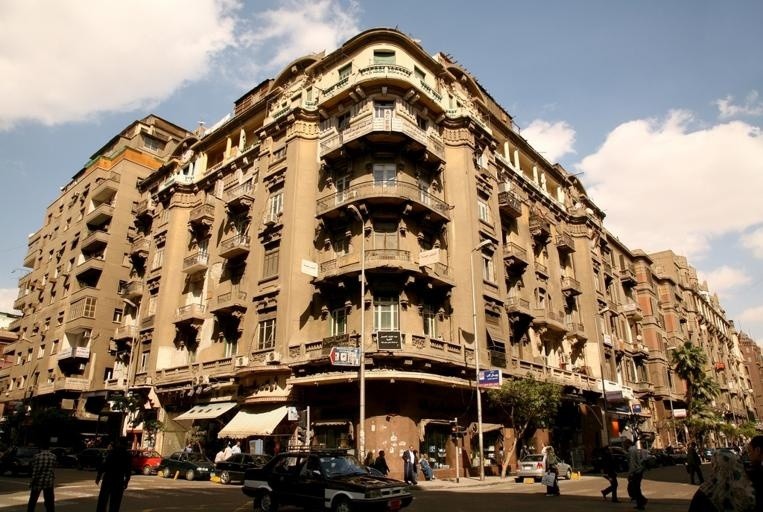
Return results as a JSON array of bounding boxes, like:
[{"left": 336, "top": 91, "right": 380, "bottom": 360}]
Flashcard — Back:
[
  {"left": 559, "top": 352, "right": 570, "bottom": 367},
  {"left": 265, "top": 351, "right": 280, "bottom": 363},
  {"left": 234, "top": 355, "right": 247, "bottom": 367}
]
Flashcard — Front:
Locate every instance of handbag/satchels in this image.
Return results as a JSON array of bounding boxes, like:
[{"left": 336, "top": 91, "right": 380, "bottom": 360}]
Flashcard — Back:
[
  {"left": 628, "top": 462, "right": 641, "bottom": 474},
  {"left": 541, "top": 471, "right": 556, "bottom": 488},
  {"left": 685, "top": 464, "right": 698, "bottom": 475}
]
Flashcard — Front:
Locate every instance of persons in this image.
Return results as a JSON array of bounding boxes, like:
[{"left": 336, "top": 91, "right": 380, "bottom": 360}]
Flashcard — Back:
[
  {"left": 185, "top": 443, "right": 193, "bottom": 453},
  {"left": 193, "top": 440, "right": 204, "bottom": 454},
  {"left": 215, "top": 442, "right": 243, "bottom": 462},
  {"left": 346, "top": 434, "right": 390, "bottom": 475},
  {"left": 687, "top": 442, "right": 704, "bottom": 484},
  {"left": 745, "top": 436, "right": 763, "bottom": 512},
  {"left": 95, "top": 436, "right": 133, "bottom": 512},
  {"left": 402, "top": 444, "right": 436, "bottom": 485},
  {"left": 601, "top": 444, "right": 622, "bottom": 504},
  {"left": 623, "top": 439, "right": 649, "bottom": 512},
  {"left": 27, "top": 442, "right": 58, "bottom": 512},
  {"left": 520, "top": 441, "right": 561, "bottom": 497}
]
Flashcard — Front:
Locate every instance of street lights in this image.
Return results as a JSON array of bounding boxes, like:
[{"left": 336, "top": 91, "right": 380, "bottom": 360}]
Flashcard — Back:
[
  {"left": 593, "top": 305, "right": 613, "bottom": 447},
  {"left": 343, "top": 203, "right": 366, "bottom": 465},
  {"left": 22, "top": 335, "right": 32, "bottom": 398},
  {"left": 664, "top": 346, "right": 679, "bottom": 447},
  {"left": 121, "top": 297, "right": 139, "bottom": 396},
  {"left": 469, "top": 237, "right": 491, "bottom": 482},
  {"left": 0, "top": 309, "right": 24, "bottom": 319},
  {"left": 725, "top": 379, "right": 738, "bottom": 428}
]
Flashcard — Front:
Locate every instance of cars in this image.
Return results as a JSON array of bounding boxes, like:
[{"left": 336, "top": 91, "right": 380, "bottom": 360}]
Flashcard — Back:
[
  {"left": 0, "top": 446, "right": 40, "bottom": 476},
  {"left": 128, "top": 449, "right": 167, "bottom": 476},
  {"left": 513, "top": 454, "right": 573, "bottom": 484},
  {"left": 213, "top": 454, "right": 273, "bottom": 485},
  {"left": 75, "top": 449, "right": 108, "bottom": 471},
  {"left": 240, "top": 451, "right": 415, "bottom": 511},
  {"left": 161, "top": 452, "right": 216, "bottom": 482},
  {"left": 589, "top": 445, "right": 715, "bottom": 473},
  {"left": 51, "top": 447, "right": 79, "bottom": 468}
]
